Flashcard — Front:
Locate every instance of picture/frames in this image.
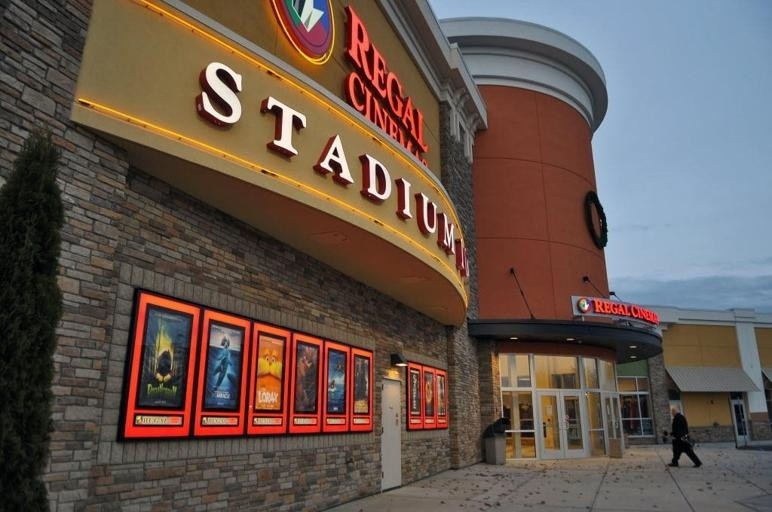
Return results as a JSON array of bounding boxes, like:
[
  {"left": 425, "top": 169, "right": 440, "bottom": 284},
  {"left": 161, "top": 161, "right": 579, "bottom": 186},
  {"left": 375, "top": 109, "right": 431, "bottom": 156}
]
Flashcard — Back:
[
  {"left": 406, "top": 359, "right": 451, "bottom": 432},
  {"left": 193, "top": 302, "right": 250, "bottom": 445},
  {"left": 322, "top": 336, "right": 350, "bottom": 437},
  {"left": 349, "top": 345, "right": 375, "bottom": 437},
  {"left": 288, "top": 331, "right": 323, "bottom": 439},
  {"left": 112, "top": 287, "right": 202, "bottom": 441},
  {"left": 246, "top": 315, "right": 291, "bottom": 442}
]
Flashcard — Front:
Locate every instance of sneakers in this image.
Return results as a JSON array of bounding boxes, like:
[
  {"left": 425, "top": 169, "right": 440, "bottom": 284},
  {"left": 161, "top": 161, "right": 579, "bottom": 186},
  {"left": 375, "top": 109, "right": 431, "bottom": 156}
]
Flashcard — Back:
[
  {"left": 668, "top": 462, "right": 678, "bottom": 467},
  {"left": 695, "top": 461, "right": 702, "bottom": 467}
]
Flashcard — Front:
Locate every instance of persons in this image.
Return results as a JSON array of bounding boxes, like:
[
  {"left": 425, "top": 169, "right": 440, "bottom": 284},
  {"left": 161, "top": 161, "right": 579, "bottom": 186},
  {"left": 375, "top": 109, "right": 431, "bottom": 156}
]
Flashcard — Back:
[
  {"left": 213, "top": 337, "right": 233, "bottom": 392},
  {"left": 668, "top": 406, "right": 702, "bottom": 467}
]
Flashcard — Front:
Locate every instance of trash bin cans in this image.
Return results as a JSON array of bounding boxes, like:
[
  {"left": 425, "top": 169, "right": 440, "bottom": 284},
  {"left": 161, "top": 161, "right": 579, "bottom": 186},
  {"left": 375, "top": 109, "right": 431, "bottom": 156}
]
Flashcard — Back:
[{"left": 482, "top": 417, "right": 509, "bottom": 465}]
